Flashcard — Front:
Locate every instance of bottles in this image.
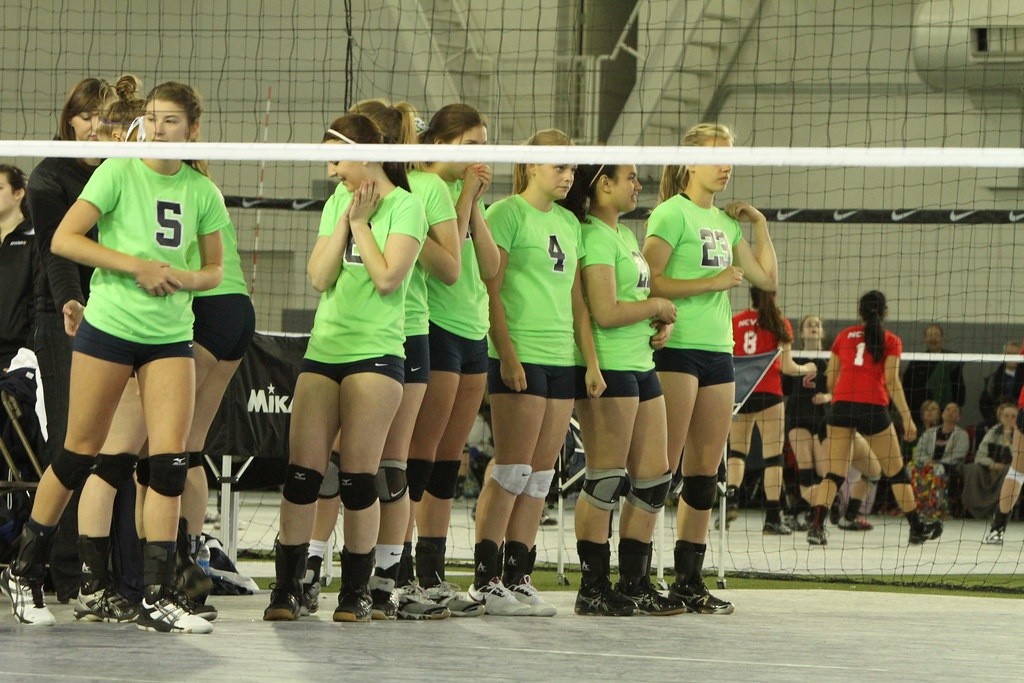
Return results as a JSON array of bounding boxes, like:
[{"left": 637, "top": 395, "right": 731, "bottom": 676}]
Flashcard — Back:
[{"left": 194, "top": 535, "right": 210, "bottom": 571}]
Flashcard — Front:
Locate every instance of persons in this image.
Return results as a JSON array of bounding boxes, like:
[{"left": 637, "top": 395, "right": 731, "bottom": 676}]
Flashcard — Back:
[
  {"left": 780, "top": 316, "right": 881, "bottom": 531},
  {"left": 714, "top": 285, "right": 817, "bottom": 535},
  {"left": 0, "top": 75, "right": 257, "bottom": 634},
  {"left": 903, "top": 323, "right": 1024, "bottom": 520},
  {"left": 614, "top": 124, "right": 778, "bottom": 613},
  {"left": 556, "top": 162, "right": 684, "bottom": 616},
  {"left": 806, "top": 289, "right": 943, "bottom": 544},
  {"left": 469, "top": 128, "right": 607, "bottom": 616},
  {"left": 264, "top": 99, "right": 557, "bottom": 622},
  {"left": 981, "top": 338, "right": 1024, "bottom": 545}
]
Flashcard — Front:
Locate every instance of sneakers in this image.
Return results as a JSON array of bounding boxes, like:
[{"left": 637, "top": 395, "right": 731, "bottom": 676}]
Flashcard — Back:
[
  {"left": 427, "top": 572, "right": 486, "bottom": 616},
  {"left": 540, "top": 500, "right": 557, "bottom": 527},
  {"left": 613, "top": 576, "right": 686, "bottom": 615},
  {"left": 763, "top": 502, "right": 874, "bottom": 545},
  {"left": 508, "top": 575, "right": 557, "bottom": 616},
  {"left": 137, "top": 593, "right": 213, "bottom": 633},
  {"left": 170, "top": 590, "right": 217, "bottom": 621},
  {"left": 667, "top": 583, "right": 736, "bottom": 615},
  {"left": 574, "top": 577, "right": 640, "bottom": 615},
  {"left": 333, "top": 582, "right": 374, "bottom": 622},
  {"left": 987, "top": 528, "right": 1005, "bottom": 544},
  {"left": 908, "top": 520, "right": 943, "bottom": 545},
  {"left": 400, "top": 580, "right": 450, "bottom": 619},
  {"left": 467, "top": 576, "right": 531, "bottom": 615},
  {"left": 75, "top": 586, "right": 139, "bottom": 624},
  {"left": 263, "top": 580, "right": 303, "bottom": 620},
  {"left": 299, "top": 581, "right": 322, "bottom": 616},
  {"left": 370, "top": 577, "right": 400, "bottom": 620},
  {"left": 1, "top": 560, "right": 57, "bottom": 626},
  {"left": 714, "top": 503, "right": 739, "bottom": 531}
]
